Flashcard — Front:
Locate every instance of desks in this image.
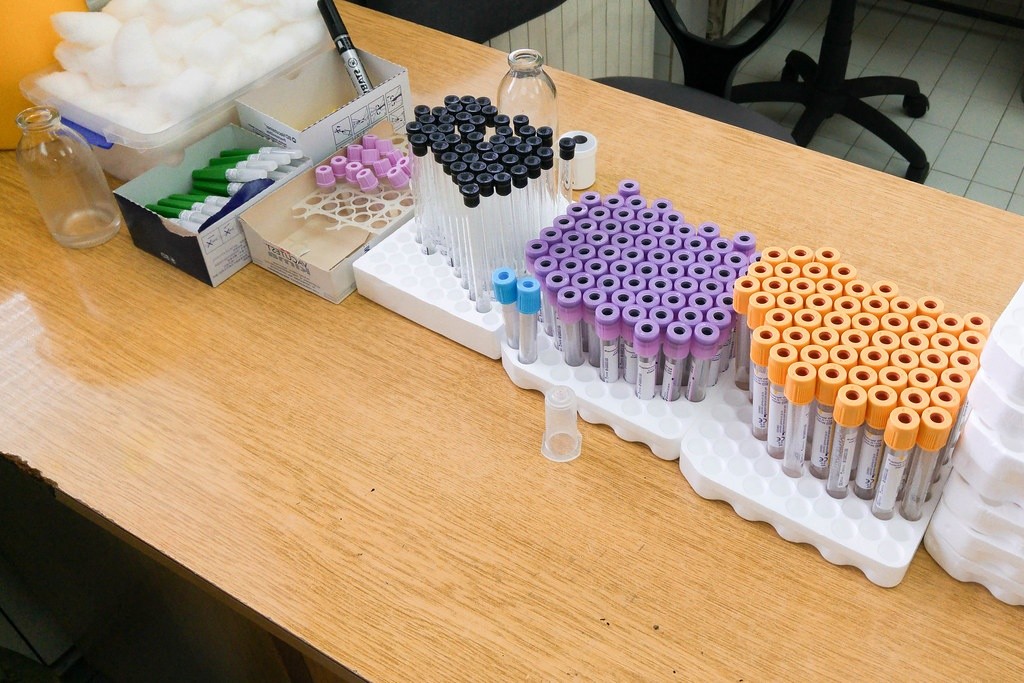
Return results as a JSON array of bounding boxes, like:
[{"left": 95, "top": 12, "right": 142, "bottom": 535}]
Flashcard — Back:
[{"left": 0, "top": 0, "right": 1024, "bottom": 683}]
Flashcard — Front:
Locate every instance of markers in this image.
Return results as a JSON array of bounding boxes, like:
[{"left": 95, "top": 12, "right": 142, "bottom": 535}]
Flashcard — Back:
[{"left": 318, "top": 0, "right": 372, "bottom": 98}]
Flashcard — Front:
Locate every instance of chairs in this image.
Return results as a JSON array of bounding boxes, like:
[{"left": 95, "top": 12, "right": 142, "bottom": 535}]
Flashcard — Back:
[{"left": 587, "top": 0, "right": 932, "bottom": 185}]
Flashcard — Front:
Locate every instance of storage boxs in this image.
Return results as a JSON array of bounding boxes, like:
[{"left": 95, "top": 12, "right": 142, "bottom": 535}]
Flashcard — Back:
[{"left": 19, "top": 17, "right": 418, "bottom": 303}]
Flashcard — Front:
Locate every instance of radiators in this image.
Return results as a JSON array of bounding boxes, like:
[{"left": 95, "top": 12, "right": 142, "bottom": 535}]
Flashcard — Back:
[{"left": 481, "top": 0, "right": 655, "bottom": 83}]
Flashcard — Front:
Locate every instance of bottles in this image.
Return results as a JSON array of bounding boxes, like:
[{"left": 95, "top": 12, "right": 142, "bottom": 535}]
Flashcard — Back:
[
  {"left": 13, "top": 105, "right": 123, "bottom": 250},
  {"left": 496, "top": 49, "right": 559, "bottom": 152}
]
